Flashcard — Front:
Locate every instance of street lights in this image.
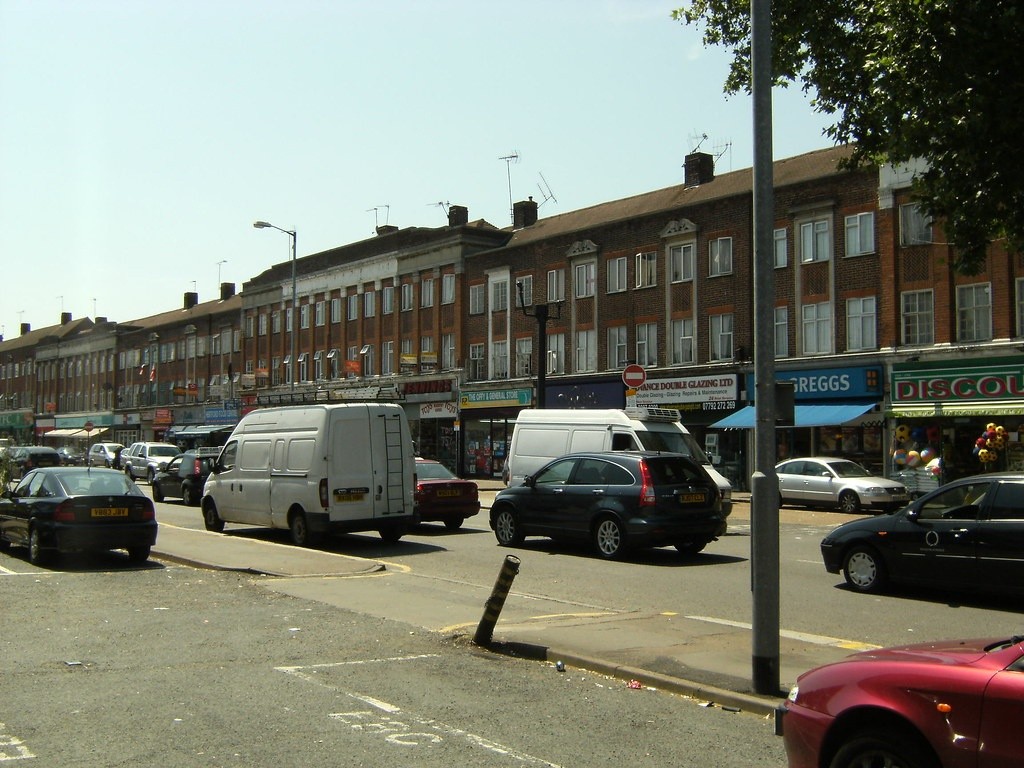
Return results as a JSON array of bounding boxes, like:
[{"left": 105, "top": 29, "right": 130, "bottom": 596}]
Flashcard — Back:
[{"left": 254, "top": 220, "right": 297, "bottom": 400}]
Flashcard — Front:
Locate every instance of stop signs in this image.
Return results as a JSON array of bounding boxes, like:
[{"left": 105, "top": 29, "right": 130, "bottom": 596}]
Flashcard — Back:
[
  {"left": 85, "top": 422, "right": 94, "bottom": 431},
  {"left": 622, "top": 365, "right": 646, "bottom": 388}
]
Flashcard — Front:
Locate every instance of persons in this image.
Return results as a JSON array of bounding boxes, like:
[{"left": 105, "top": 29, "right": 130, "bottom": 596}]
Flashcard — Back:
[{"left": 111, "top": 446, "right": 123, "bottom": 470}]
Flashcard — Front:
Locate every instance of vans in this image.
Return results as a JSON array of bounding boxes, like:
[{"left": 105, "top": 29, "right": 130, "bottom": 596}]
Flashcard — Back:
[
  {"left": 201, "top": 401, "right": 418, "bottom": 545},
  {"left": 507, "top": 406, "right": 733, "bottom": 515}
]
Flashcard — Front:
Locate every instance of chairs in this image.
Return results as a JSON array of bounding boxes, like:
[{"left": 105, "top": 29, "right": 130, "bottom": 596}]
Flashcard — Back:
[{"left": 580, "top": 468, "right": 604, "bottom": 484}]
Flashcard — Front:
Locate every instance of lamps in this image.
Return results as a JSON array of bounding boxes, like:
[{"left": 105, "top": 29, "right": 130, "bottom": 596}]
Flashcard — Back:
[{"left": 907, "top": 355, "right": 921, "bottom": 363}]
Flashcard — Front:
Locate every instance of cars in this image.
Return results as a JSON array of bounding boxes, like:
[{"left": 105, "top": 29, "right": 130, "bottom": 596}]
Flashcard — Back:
[
  {"left": 88, "top": 439, "right": 126, "bottom": 467},
  {"left": 410, "top": 457, "right": 480, "bottom": 530},
  {"left": 57, "top": 445, "right": 86, "bottom": 466},
  {"left": 10, "top": 446, "right": 62, "bottom": 480},
  {"left": 152, "top": 451, "right": 229, "bottom": 505},
  {"left": 819, "top": 471, "right": 1024, "bottom": 592},
  {"left": 119, "top": 448, "right": 129, "bottom": 466},
  {"left": 773, "top": 457, "right": 909, "bottom": 513},
  {"left": 0, "top": 466, "right": 158, "bottom": 567},
  {"left": 772, "top": 633, "right": 1024, "bottom": 768},
  {"left": 0, "top": 439, "right": 14, "bottom": 461},
  {"left": 488, "top": 451, "right": 727, "bottom": 558}
]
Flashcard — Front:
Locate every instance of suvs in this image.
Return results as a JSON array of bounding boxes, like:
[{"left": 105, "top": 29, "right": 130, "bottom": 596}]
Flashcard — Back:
[{"left": 125, "top": 443, "right": 185, "bottom": 485}]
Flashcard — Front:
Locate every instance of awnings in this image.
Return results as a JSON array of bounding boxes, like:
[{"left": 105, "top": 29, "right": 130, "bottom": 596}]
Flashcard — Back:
[
  {"left": 706, "top": 402, "right": 877, "bottom": 429},
  {"left": 164, "top": 425, "right": 235, "bottom": 437},
  {"left": 38, "top": 428, "right": 110, "bottom": 438}
]
